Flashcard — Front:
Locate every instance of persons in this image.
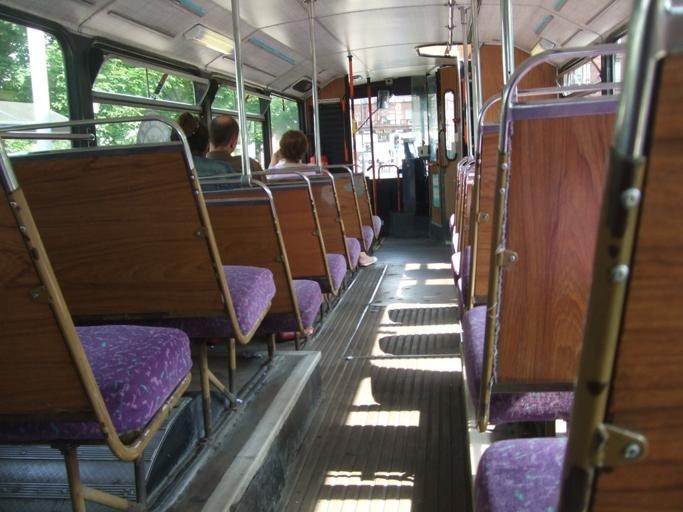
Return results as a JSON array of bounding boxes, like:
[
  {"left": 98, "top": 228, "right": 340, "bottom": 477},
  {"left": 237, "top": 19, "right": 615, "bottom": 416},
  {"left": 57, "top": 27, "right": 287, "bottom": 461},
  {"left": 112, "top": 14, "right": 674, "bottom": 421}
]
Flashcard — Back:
[
  {"left": 170, "top": 112, "right": 241, "bottom": 190},
  {"left": 205, "top": 115, "right": 267, "bottom": 184},
  {"left": 266, "top": 130, "right": 378, "bottom": 267}
]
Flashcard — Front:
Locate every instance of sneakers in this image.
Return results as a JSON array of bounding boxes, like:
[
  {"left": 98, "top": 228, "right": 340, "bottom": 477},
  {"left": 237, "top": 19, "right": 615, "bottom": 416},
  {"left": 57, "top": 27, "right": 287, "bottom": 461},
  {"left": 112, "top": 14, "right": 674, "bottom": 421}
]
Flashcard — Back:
[
  {"left": 274, "top": 326, "right": 318, "bottom": 342},
  {"left": 357, "top": 249, "right": 379, "bottom": 268}
]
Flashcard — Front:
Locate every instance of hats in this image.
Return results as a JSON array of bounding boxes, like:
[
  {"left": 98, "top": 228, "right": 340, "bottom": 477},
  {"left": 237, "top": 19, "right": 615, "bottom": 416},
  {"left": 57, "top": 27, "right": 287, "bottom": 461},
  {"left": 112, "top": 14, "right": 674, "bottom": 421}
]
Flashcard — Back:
[{"left": 171, "top": 111, "right": 202, "bottom": 139}]
